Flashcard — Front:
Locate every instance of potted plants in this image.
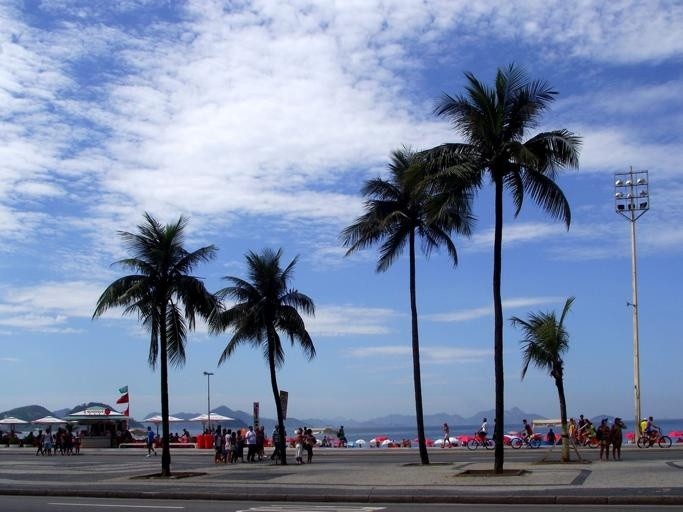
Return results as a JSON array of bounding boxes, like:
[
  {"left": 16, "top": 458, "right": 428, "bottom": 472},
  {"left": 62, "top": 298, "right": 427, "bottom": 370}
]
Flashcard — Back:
[{"left": 0, "top": 436, "right": 33, "bottom": 448}]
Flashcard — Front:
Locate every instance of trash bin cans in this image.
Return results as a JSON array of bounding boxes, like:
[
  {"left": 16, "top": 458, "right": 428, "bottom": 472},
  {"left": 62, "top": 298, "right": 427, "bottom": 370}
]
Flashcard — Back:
[{"left": 196, "top": 434, "right": 213, "bottom": 449}]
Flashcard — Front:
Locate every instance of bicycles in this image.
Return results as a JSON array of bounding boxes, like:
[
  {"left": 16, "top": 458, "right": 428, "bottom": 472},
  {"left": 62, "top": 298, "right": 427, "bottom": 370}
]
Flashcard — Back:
[
  {"left": 466, "top": 432, "right": 495, "bottom": 450},
  {"left": 637, "top": 427, "right": 673, "bottom": 448},
  {"left": 568, "top": 428, "right": 600, "bottom": 450},
  {"left": 509, "top": 429, "right": 542, "bottom": 450}
]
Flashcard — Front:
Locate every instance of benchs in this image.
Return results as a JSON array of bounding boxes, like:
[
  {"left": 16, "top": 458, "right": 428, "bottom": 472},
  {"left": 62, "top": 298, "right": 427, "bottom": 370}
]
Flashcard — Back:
[{"left": 119, "top": 443, "right": 198, "bottom": 449}]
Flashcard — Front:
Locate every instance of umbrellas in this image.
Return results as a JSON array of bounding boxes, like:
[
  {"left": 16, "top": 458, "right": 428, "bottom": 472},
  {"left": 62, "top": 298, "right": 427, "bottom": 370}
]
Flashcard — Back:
[
  {"left": 135, "top": 414, "right": 183, "bottom": 435},
  {"left": 187, "top": 411, "right": 235, "bottom": 425},
  {"left": 0, "top": 415, "right": 28, "bottom": 434},
  {"left": 667, "top": 430, "right": 683, "bottom": 439},
  {"left": 30, "top": 414, "right": 65, "bottom": 429},
  {"left": 622, "top": 432, "right": 634, "bottom": 439},
  {"left": 285, "top": 430, "right": 564, "bottom": 447}
]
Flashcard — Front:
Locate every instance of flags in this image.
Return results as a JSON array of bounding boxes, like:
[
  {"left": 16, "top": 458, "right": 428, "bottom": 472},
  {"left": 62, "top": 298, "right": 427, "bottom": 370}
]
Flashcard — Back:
[
  {"left": 118, "top": 384, "right": 127, "bottom": 394},
  {"left": 116, "top": 393, "right": 128, "bottom": 403},
  {"left": 122, "top": 405, "right": 129, "bottom": 416}
]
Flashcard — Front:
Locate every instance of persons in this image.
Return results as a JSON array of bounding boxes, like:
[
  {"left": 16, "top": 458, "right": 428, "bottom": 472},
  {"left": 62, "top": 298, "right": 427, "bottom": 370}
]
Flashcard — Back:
[
  {"left": 644, "top": 416, "right": 661, "bottom": 441},
  {"left": 302, "top": 429, "right": 315, "bottom": 463},
  {"left": 205, "top": 424, "right": 265, "bottom": 464},
  {"left": 269, "top": 424, "right": 281, "bottom": 460},
  {"left": 474, "top": 417, "right": 488, "bottom": 449},
  {"left": 640, "top": 417, "right": 648, "bottom": 444},
  {"left": 276, "top": 425, "right": 287, "bottom": 458},
  {"left": 32, "top": 426, "right": 81, "bottom": 456},
  {"left": 439, "top": 421, "right": 451, "bottom": 449},
  {"left": 546, "top": 427, "right": 556, "bottom": 444},
  {"left": 169, "top": 432, "right": 173, "bottom": 441},
  {"left": 174, "top": 432, "right": 178, "bottom": 440},
  {"left": 293, "top": 429, "right": 303, "bottom": 464},
  {"left": 568, "top": 413, "right": 627, "bottom": 462},
  {"left": 336, "top": 424, "right": 347, "bottom": 448},
  {"left": 182, "top": 429, "right": 190, "bottom": 443},
  {"left": 144, "top": 425, "right": 156, "bottom": 458},
  {"left": 519, "top": 419, "right": 533, "bottom": 446}
]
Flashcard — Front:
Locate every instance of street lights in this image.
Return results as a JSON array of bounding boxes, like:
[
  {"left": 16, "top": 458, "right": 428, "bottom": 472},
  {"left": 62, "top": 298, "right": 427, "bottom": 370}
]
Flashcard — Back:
[
  {"left": 612, "top": 165, "right": 652, "bottom": 446},
  {"left": 202, "top": 371, "right": 214, "bottom": 430}
]
